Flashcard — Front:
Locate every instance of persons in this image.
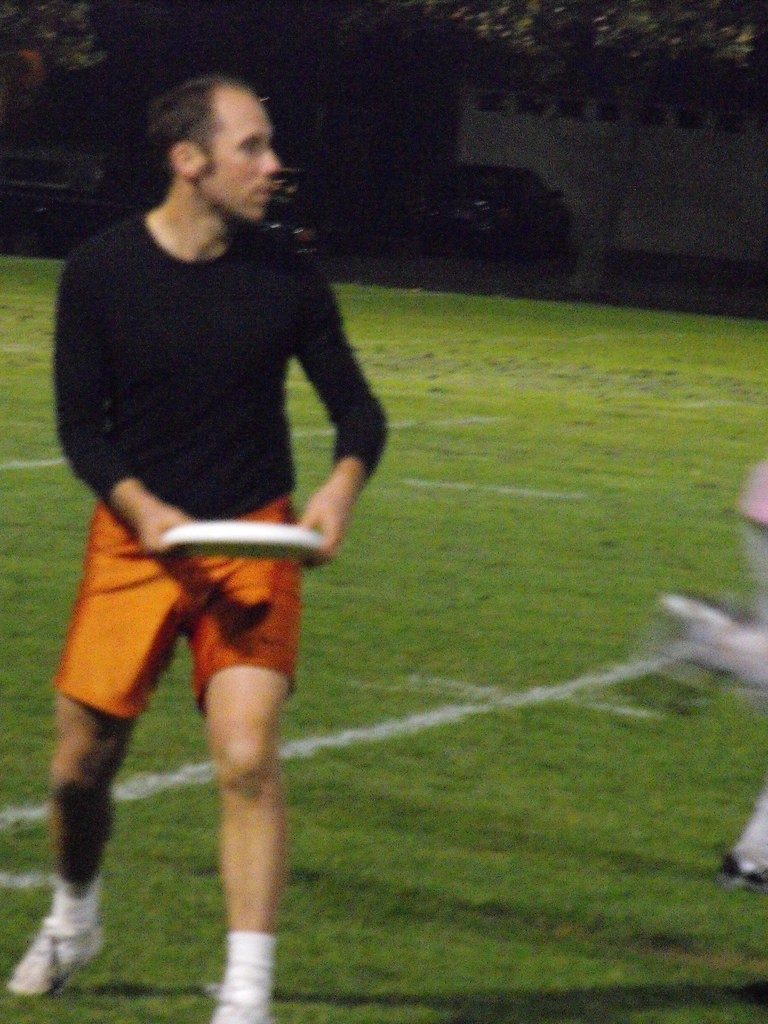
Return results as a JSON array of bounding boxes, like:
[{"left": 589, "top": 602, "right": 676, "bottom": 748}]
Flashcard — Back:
[
  {"left": 652, "top": 459, "right": 768, "bottom": 892},
  {"left": 0, "top": 71, "right": 390, "bottom": 1023}
]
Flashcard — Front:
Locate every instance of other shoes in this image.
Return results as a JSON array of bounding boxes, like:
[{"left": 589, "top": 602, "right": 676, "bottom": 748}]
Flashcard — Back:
[
  {"left": 5, "top": 921, "right": 102, "bottom": 998},
  {"left": 208, "top": 977, "right": 273, "bottom": 1024},
  {"left": 720, "top": 850, "right": 768, "bottom": 893}
]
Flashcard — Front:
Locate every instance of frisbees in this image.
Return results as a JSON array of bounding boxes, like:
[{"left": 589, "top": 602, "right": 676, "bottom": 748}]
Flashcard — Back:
[{"left": 155, "top": 521, "right": 322, "bottom": 561}]
